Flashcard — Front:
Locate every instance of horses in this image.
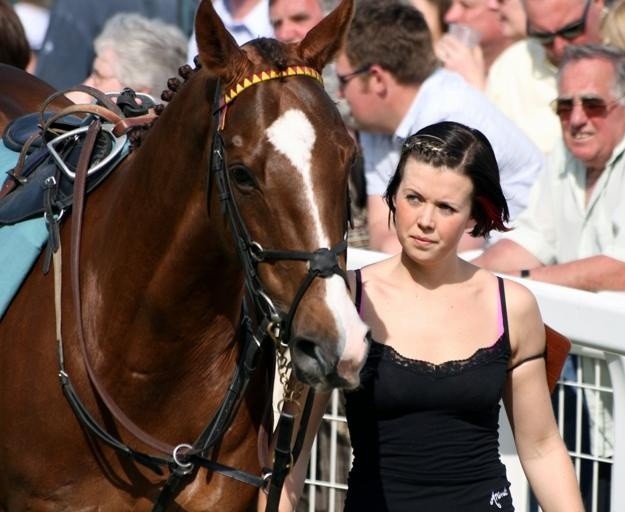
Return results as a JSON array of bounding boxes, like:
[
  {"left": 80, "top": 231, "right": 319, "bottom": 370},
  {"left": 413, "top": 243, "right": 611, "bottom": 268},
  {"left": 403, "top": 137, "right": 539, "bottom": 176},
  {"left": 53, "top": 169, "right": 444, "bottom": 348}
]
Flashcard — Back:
[{"left": 0, "top": 0, "right": 371, "bottom": 512}]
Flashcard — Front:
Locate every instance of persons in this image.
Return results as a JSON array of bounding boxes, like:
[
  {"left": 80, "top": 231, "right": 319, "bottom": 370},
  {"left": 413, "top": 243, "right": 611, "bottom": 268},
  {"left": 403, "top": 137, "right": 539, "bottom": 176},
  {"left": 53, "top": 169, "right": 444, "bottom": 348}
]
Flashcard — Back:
[{"left": 2, "top": 1, "right": 625, "bottom": 511}]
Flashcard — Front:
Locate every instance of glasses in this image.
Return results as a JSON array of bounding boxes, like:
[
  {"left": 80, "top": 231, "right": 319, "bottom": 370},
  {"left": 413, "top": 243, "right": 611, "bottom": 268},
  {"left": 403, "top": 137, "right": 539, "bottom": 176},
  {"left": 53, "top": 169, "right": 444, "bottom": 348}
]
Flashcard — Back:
[
  {"left": 550, "top": 98, "right": 620, "bottom": 118},
  {"left": 336, "top": 63, "right": 370, "bottom": 85},
  {"left": 526, "top": 1, "right": 590, "bottom": 45}
]
